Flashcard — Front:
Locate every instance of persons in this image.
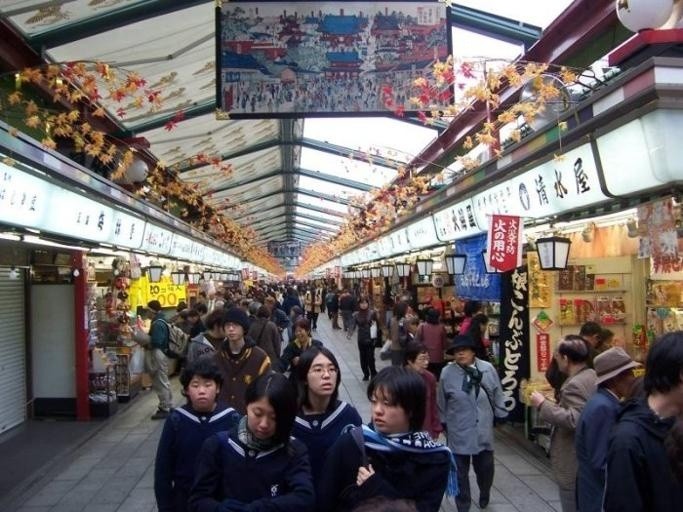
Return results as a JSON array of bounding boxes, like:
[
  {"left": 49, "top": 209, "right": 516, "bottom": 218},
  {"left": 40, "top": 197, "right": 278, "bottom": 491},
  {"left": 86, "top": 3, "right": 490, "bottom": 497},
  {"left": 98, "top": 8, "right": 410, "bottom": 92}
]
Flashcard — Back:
[
  {"left": 574, "top": 345, "right": 643, "bottom": 512},
  {"left": 144, "top": 278, "right": 509, "bottom": 512},
  {"left": 607, "top": 329, "right": 683, "bottom": 511},
  {"left": 220, "top": 7, "right": 451, "bottom": 112},
  {"left": 530, "top": 335, "right": 599, "bottom": 512},
  {"left": 545, "top": 321, "right": 607, "bottom": 394}
]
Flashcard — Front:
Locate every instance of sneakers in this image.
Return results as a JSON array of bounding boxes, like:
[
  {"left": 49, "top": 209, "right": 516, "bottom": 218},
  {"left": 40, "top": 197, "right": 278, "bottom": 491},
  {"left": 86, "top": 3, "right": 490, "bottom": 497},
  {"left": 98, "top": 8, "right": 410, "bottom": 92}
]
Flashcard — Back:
[
  {"left": 362, "top": 375, "right": 370, "bottom": 381},
  {"left": 477, "top": 487, "right": 491, "bottom": 509},
  {"left": 150, "top": 410, "right": 170, "bottom": 419}
]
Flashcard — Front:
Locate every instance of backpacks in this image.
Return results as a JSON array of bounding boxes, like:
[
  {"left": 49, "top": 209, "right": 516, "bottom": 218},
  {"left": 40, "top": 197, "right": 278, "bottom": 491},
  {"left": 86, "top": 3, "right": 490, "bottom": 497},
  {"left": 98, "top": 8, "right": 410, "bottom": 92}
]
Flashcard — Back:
[
  {"left": 396, "top": 318, "right": 410, "bottom": 349},
  {"left": 168, "top": 325, "right": 188, "bottom": 354}
]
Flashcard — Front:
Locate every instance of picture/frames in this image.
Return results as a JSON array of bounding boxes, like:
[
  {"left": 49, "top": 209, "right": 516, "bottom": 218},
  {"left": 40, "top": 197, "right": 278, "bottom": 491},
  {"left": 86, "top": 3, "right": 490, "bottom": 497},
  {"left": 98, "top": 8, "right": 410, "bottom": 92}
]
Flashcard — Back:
[
  {"left": 268, "top": 240, "right": 301, "bottom": 257},
  {"left": 215, "top": 1, "right": 454, "bottom": 120}
]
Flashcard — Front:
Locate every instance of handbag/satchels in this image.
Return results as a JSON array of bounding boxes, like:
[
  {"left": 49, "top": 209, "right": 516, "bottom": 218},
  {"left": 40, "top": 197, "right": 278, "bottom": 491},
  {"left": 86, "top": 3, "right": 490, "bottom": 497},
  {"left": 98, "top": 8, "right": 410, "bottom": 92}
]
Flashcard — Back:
[{"left": 369, "top": 320, "right": 378, "bottom": 342}]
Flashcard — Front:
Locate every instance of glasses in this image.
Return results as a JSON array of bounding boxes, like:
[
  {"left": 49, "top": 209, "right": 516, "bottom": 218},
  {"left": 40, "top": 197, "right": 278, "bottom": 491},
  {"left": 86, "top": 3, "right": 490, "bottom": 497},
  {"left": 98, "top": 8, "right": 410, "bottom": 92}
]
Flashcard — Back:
[
  {"left": 307, "top": 366, "right": 339, "bottom": 376},
  {"left": 224, "top": 322, "right": 241, "bottom": 328}
]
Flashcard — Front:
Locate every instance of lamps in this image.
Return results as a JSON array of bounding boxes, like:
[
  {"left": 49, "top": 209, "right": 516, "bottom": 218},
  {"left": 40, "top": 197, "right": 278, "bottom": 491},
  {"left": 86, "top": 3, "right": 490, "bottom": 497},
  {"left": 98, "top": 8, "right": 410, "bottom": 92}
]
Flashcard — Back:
[
  {"left": 445, "top": 255, "right": 467, "bottom": 275},
  {"left": 344, "top": 265, "right": 394, "bottom": 279},
  {"left": 396, "top": 263, "right": 411, "bottom": 277},
  {"left": 534, "top": 222, "right": 572, "bottom": 270},
  {"left": 616, "top": 0, "right": 675, "bottom": 31},
  {"left": 171, "top": 272, "right": 186, "bottom": 284},
  {"left": 188, "top": 271, "right": 239, "bottom": 285},
  {"left": 481, "top": 249, "right": 505, "bottom": 273},
  {"left": 416, "top": 259, "right": 433, "bottom": 276},
  {"left": 149, "top": 266, "right": 163, "bottom": 282}
]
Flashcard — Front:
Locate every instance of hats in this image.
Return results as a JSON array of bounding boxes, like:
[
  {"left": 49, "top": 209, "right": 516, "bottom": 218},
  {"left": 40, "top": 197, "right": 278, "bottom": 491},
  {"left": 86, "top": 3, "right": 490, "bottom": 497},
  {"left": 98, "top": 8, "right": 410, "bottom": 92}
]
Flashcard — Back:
[
  {"left": 592, "top": 345, "right": 640, "bottom": 386},
  {"left": 445, "top": 334, "right": 482, "bottom": 356},
  {"left": 222, "top": 307, "right": 251, "bottom": 337}
]
